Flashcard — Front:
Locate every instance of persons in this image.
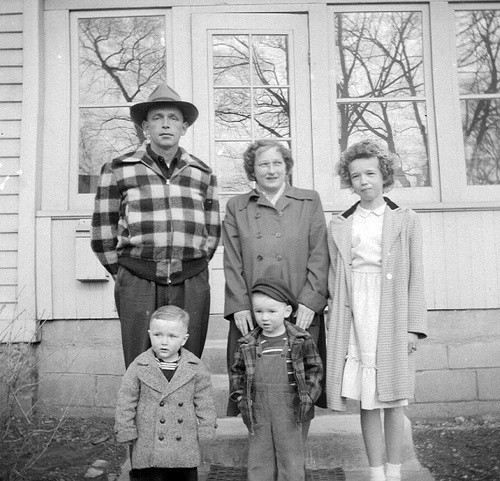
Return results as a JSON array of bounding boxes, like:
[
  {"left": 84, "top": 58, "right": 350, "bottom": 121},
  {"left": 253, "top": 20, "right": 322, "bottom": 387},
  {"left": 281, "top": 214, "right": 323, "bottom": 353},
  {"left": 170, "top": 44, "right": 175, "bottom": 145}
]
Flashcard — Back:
[
  {"left": 326, "top": 140, "right": 428, "bottom": 481},
  {"left": 223, "top": 140, "right": 329, "bottom": 417},
  {"left": 229, "top": 278, "right": 323, "bottom": 481},
  {"left": 114, "top": 305, "right": 219, "bottom": 481},
  {"left": 90, "top": 82, "right": 222, "bottom": 370}
]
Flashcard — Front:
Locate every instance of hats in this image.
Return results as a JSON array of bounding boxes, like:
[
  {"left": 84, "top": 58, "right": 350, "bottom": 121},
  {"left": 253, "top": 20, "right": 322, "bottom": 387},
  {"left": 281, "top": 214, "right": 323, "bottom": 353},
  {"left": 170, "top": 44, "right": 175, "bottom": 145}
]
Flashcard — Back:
[
  {"left": 251, "top": 278, "right": 298, "bottom": 313},
  {"left": 130, "top": 83, "right": 199, "bottom": 129}
]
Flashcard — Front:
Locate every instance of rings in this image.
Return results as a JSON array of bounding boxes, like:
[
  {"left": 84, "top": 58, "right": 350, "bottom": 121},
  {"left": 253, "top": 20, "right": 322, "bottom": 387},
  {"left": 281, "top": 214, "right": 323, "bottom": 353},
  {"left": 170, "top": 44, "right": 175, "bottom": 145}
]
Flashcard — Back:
[{"left": 412, "top": 347, "right": 416, "bottom": 349}]
